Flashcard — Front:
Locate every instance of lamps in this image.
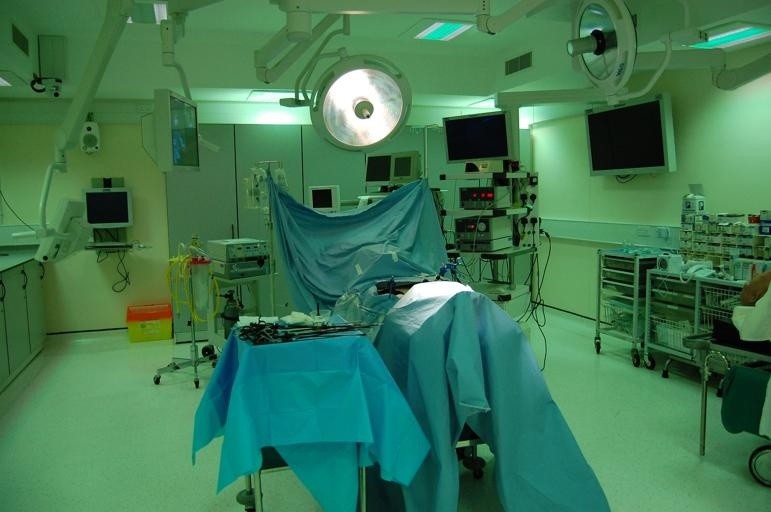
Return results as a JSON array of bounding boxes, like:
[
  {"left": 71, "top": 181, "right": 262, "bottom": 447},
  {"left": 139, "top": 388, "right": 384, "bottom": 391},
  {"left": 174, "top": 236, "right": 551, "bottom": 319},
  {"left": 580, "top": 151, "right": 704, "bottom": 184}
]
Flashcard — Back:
[
  {"left": 311, "top": 57, "right": 415, "bottom": 155},
  {"left": 564, "top": 1, "right": 640, "bottom": 101}
]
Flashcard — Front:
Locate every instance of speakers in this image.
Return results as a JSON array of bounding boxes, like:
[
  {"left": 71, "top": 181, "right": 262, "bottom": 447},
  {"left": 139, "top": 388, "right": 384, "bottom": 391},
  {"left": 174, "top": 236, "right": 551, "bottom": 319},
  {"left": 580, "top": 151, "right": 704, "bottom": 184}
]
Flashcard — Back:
[{"left": 80, "top": 122, "right": 100, "bottom": 156}]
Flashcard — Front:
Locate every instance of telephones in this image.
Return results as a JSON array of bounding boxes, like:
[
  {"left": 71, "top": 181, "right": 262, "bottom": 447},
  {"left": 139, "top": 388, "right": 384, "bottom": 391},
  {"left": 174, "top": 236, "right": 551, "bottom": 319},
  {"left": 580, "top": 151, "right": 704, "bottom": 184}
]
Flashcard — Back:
[{"left": 680, "top": 262, "right": 716, "bottom": 284}]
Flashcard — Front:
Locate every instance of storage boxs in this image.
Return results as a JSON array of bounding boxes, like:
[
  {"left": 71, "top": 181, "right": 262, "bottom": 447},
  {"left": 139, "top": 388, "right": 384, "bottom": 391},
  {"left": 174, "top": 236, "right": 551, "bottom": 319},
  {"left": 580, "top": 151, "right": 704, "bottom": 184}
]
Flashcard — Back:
[{"left": 126, "top": 302, "right": 174, "bottom": 345}]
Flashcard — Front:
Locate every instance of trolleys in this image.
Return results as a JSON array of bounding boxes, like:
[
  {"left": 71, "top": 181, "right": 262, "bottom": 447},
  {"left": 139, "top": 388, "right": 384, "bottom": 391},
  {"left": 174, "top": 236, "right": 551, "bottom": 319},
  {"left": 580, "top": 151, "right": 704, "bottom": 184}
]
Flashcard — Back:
[
  {"left": 643, "top": 268, "right": 715, "bottom": 383},
  {"left": 696, "top": 261, "right": 771, "bottom": 397},
  {"left": 593, "top": 247, "right": 663, "bottom": 367},
  {"left": 237, "top": 311, "right": 367, "bottom": 512}
]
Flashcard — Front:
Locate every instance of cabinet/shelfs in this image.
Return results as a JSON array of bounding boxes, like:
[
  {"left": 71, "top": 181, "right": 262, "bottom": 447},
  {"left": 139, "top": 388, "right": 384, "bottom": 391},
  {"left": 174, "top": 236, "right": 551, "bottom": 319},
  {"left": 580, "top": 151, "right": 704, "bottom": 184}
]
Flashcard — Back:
[{"left": 0, "top": 248, "right": 49, "bottom": 392}]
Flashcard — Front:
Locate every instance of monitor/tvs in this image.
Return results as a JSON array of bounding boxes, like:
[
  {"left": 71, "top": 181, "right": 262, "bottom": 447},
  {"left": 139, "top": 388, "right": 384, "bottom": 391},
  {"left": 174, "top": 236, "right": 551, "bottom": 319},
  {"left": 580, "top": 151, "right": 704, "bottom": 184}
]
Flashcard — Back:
[
  {"left": 442, "top": 110, "right": 512, "bottom": 165},
  {"left": 391, "top": 151, "right": 423, "bottom": 183},
  {"left": 83, "top": 188, "right": 134, "bottom": 228},
  {"left": 362, "top": 153, "right": 393, "bottom": 195},
  {"left": 141, "top": 88, "right": 200, "bottom": 172},
  {"left": 583, "top": 91, "right": 677, "bottom": 177},
  {"left": 309, "top": 185, "right": 340, "bottom": 213}
]
